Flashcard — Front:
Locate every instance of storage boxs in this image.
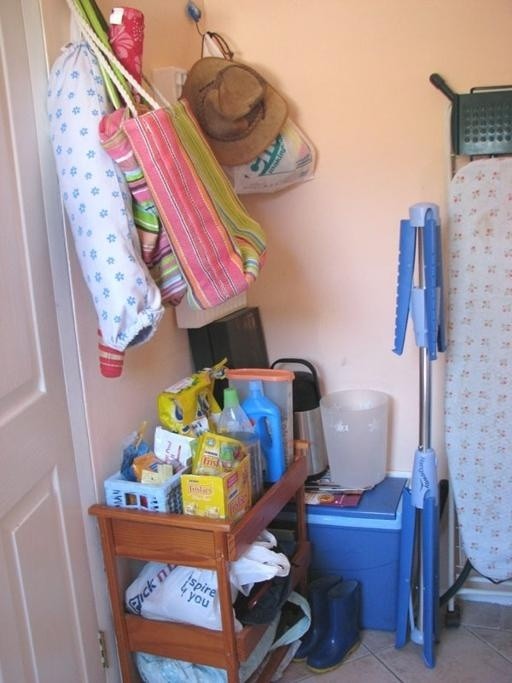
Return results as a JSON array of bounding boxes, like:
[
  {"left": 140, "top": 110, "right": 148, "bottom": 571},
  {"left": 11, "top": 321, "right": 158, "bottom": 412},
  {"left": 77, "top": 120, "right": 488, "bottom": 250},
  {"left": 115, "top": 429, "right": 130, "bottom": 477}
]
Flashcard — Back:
[{"left": 269, "top": 469, "right": 410, "bottom": 650}]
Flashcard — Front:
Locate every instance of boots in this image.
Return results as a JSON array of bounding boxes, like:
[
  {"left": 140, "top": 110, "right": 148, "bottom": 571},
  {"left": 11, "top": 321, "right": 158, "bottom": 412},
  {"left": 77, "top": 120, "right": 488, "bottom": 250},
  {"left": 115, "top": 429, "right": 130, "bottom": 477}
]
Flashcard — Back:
[
  {"left": 305, "top": 580, "right": 361, "bottom": 675},
  {"left": 294, "top": 575, "right": 343, "bottom": 663}
]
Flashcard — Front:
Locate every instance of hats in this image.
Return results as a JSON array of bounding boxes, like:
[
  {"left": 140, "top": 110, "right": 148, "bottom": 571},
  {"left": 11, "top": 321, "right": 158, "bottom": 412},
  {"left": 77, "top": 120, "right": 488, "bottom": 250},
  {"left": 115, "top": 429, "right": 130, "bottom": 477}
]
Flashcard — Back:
[{"left": 181, "top": 56, "right": 290, "bottom": 169}]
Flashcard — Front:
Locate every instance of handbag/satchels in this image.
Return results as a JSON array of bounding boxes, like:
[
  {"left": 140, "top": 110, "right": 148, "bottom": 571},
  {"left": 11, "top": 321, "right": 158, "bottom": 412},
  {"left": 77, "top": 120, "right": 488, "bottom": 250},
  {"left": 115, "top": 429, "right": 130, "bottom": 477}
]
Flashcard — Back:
[{"left": 99, "top": 95, "right": 268, "bottom": 315}]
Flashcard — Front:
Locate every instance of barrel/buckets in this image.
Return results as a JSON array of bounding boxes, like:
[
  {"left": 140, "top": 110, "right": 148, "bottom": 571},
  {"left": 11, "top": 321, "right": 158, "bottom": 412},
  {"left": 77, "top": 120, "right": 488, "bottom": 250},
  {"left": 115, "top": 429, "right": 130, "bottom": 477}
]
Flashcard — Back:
[{"left": 321, "top": 390, "right": 388, "bottom": 490}]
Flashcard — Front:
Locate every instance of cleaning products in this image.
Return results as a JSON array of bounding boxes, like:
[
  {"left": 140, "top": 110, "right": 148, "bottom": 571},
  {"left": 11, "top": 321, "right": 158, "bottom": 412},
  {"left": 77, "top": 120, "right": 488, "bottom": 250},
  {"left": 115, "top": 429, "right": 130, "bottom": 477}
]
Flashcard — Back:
[
  {"left": 217, "top": 389, "right": 266, "bottom": 481},
  {"left": 241, "top": 379, "right": 284, "bottom": 483}
]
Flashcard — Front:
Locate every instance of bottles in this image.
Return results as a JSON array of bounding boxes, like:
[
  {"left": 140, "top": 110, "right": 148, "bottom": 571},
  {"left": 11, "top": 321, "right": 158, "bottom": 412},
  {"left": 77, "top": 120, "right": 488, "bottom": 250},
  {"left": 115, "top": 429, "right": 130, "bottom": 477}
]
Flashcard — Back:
[{"left": 271, "top": 359, "right": 329, "bottom": 481}]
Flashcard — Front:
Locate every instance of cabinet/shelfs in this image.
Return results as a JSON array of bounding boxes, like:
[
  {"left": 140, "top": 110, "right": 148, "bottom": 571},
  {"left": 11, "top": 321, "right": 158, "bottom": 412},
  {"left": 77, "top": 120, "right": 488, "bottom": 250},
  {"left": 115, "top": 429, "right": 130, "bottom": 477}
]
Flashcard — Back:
[{"left": 88, "top": 442, "right": 312, "bottom": 683}]
